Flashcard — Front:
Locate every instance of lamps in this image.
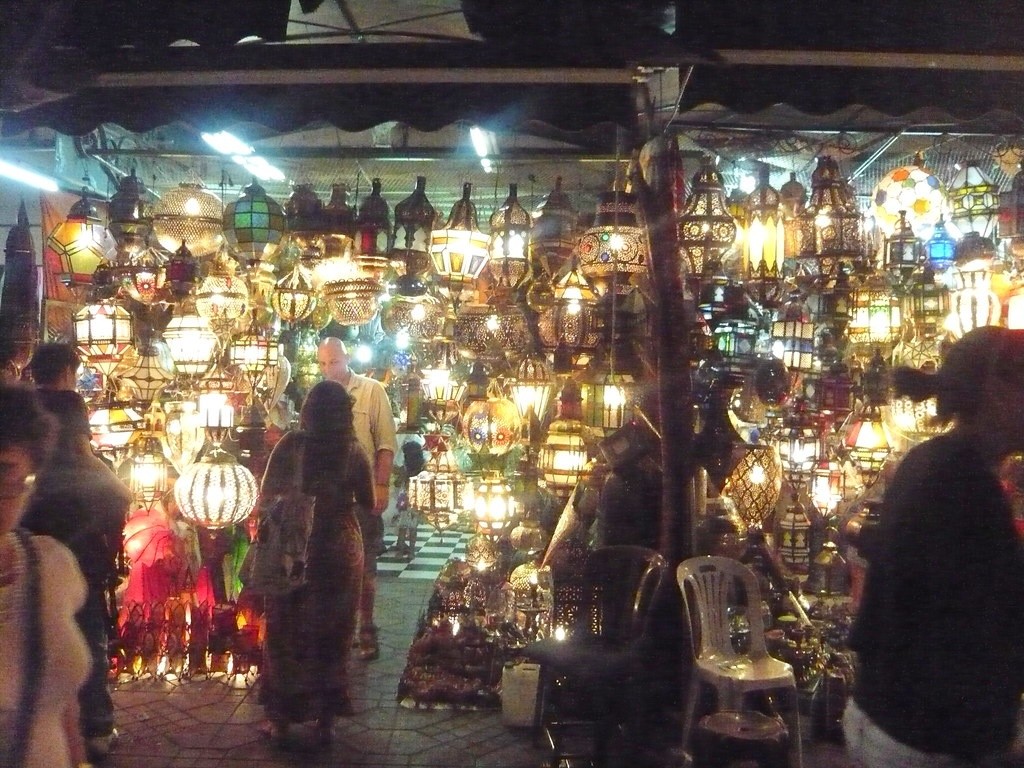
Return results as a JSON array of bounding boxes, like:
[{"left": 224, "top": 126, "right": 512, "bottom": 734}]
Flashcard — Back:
[{"left": 45, "top": 134, "right": 1024, "bottom": 692}]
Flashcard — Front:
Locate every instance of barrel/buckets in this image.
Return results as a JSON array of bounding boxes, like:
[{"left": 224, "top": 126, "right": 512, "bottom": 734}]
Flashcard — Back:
[{"left": 501, "top": 662, "right": 540, "bottom": 725}]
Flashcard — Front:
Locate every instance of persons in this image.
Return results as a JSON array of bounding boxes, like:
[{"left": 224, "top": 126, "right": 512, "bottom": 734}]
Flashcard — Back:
[
  {"left": 26, "top": 342, "right": 81, "bottom": 391},
  {"left": 0, "top": 386, "right": 93, "bottom": 768},
  {"left": 318, "top": 337, "right": 398, "bottom": 659},
  {"left": 841, "top": 326, "right": 1024, "bottom": 768},
  {"left": 257, "top": 379, "right": 377, "bottom": 743},
  {"left": 394, "top": 441, "right": 426, "bottom": 559},
  {"left": 19, "top": 390, "right": 130, "bottom": 756}
]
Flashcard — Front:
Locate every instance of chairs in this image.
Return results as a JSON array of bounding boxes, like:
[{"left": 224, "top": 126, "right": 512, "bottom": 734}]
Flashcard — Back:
[
  {"left": 522, "top": 543, "right": 672, "bottom": 768},
  {"left": 675, "top": 556, "right": 804, "bottom": 768}
]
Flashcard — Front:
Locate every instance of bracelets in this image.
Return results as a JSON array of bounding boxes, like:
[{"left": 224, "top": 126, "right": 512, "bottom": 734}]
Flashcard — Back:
[{"left": 376, "top": 483, "right": 390, "bottom": 488}]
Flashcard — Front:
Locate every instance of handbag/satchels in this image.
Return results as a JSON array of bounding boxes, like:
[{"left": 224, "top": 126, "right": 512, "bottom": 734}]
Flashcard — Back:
[{"left": 239, "top": 438, "right": 314, "bottom": 591}]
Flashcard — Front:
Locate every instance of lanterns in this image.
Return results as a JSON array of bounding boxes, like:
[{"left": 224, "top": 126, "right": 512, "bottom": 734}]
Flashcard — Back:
[
  {"left": 174, "top": 457, "right": 259, "bottom": 528},
  {"left": 46, "top": 156, "right": 1000, "bottom": 569}
]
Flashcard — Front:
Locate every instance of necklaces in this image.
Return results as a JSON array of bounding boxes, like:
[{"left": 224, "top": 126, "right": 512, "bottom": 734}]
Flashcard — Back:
[{"left": 0, "top": 533, "right": 19, "bottom": 578}]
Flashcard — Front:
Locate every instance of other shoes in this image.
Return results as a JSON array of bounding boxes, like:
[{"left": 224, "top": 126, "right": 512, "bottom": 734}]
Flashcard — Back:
[
  {"left": 316, "top": 720, "right": 335, "bottom": 743},
  {"left": 270, "top": 724, "right": 291, "bottom": 742},
  {"left": 358, "top": 626, "right": 378, "bottom": 658},
  {"left": 86, "top": 727, "right": 119, "bottom": 757}
]
